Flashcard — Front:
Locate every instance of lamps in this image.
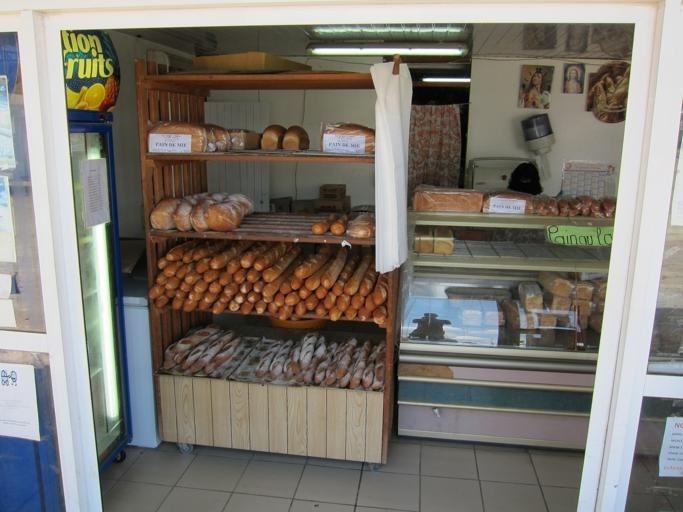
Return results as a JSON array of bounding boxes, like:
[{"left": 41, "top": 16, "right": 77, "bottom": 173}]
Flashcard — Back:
[{"left": 308, "top": 43, "right": 468, "bottom": 55}]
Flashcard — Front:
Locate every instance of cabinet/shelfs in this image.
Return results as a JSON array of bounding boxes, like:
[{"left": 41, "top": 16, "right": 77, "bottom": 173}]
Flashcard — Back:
[
  {"left": 397, "top": 208, "right": 682, "bottom": 457},
  {"left": 135, "top": 58, "right": 403, "bottom": 466}
]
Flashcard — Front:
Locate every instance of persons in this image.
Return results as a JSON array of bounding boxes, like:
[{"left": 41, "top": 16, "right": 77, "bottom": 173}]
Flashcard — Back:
[
  {"left": 520, "top": 71, "right": 550, "bottom": 109},
  {"left": 565, "top": 67, "right": 583, "bottom": 94}
]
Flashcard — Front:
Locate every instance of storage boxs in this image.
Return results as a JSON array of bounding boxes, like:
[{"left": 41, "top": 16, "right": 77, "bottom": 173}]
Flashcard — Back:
[
  {"left": 271, "top": 197, "right": 291, "bottom": 212},
  {"left": 320, "top": 184, "right": 346, "bottom": 201},
  {"left": 291, "top": 200, "right": 312, "bottom": 213},
  {"left": 353, "top": 205, "right": 374, "bottom": 212},
  {"left": 313, "top": 197, "right": 351, "bottom": 214}
]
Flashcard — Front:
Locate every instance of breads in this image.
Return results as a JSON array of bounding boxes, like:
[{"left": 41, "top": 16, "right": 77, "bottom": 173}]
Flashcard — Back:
[
  {"left": 163, "top": 324, "right": 386, "bottom": 391},
  {"left": 444, "top": 271, "right": 608, "bottom": 349},
  {"left": 330, "top": 211, "right": 349, "bottom": 236},
  {"left": 282, "top": 125, "right": 309, "bottom": 151},
  {"left": 412, "top": 227, "right": 456, "bottom": 256},
  {"left": 346, "top": 212, "right": 375, "bottom": 239},
  {"left": 312, "top": 213, "right": 336, "bottom": 235},
  {"left": 411, "top": 183, "right": 484, "bottom": 214},
  {"left": 146, "top": 121, "right": 230, "bottom": 154},
  {"left": 320, "top": 122, "right": 375, "bottom": 154},
  {"left": 149, "top": 190, "right": 255, "bottom": 232},
  {"left": 258, "top": 124, "right": 287, "bottom": 150},
  {"left": 534, "top": 193, "right": 616, "bottom": 218},
  {"left": 227, "top": 128, "right": 259, "bottom": 150},
  {"left": 481, "top": 191, "right": 535, "bottom": 215},
  {"left": 148, "top": 238, "right": 389, "bottom": 325}
]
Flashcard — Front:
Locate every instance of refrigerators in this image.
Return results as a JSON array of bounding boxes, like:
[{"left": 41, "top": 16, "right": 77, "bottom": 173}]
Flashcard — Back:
[
  {"left": 1, "top": 30, "right": 131, "bottom": 511},
  {"left": 120, "top": 269, "right": 163, "bottom": 449}
]
